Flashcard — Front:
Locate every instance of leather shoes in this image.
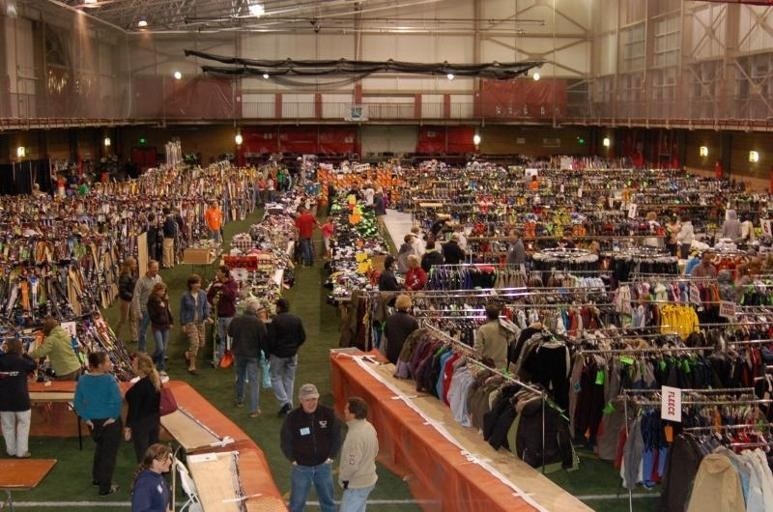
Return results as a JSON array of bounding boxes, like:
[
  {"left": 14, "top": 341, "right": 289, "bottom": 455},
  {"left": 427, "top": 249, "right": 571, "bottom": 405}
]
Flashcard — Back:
[
  {"left": 183, "top": 351, "right": 199, "bottom": 375},
  {"left": 279, "top": 403, "right": 292, "bottom": 415}
]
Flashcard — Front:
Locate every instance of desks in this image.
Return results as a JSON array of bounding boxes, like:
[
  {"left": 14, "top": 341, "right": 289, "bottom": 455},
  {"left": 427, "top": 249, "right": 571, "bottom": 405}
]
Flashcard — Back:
[
  {"left": 0, "top": 459, "right": 57, "bottom": 512},
  {"left": 330, "top": 347, "right": 598, "bottom": 512},
  {"left": 0, "top": 379, "right": 289, "bottom": 512}
]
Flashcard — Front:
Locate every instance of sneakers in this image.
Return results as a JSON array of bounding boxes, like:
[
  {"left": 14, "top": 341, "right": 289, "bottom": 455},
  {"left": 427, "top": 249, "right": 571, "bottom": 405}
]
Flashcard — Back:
[
  {"left": 237, "top": 403, "right": 260, "bottom": 418},
  {"left": 16, "top": 452, "right": 31, "bottom": 458},
  {"left": 91, "top": 480, "right": 121, "bottom": 498}
]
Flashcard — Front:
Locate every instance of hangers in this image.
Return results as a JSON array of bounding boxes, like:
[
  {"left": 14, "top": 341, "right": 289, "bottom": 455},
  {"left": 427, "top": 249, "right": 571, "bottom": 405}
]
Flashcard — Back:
[{"left": 0, "top": 156, "right": 260, "bottom": 280}]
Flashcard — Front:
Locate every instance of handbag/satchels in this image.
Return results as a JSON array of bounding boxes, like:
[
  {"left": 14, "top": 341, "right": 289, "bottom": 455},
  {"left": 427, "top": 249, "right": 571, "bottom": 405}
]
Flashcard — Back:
[
  {"left": 73, "top": 345, "right": 84, "bottom": 363},
  {"left": 158, "top": 387, "right": 177, "bottom": 416}
]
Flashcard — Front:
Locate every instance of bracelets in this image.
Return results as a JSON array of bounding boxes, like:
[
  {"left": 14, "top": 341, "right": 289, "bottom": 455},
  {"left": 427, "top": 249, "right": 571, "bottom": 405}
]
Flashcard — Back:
[{"left": 326, "top": 457, "right": 334, "bottom": 465}]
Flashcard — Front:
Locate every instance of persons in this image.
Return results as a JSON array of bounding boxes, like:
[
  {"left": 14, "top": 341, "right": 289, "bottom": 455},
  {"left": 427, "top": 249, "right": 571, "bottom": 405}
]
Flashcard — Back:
[
  {"left": 0, "top": 339, "right": 37, "bottom": 458},
  {"left": 203, "top": 201, "right": 222, "bottom": 243},
  {"left": 379, "top": 225, "right": 465, "bottom": 290},
  {"left": 384, "top": 295, "right": 418, "bottom": 364},
  {"left": 31, "top": 172, "right": 88, "bottom": 199},
  {"left": 646, "top": 212, "right": 693, "bottom": 259},
  {"left": 347, "top": 180, "right": 385, "bottom": 217},
  {"left": 257, "top": 162, "right": 293, "bottom": 207},
  {"left": 139, "top": 208, "right": 189, "bottom": 269},
  {"left": 115, "top": 258, "right": 306, "bottom": 418},
  {"left": 723, "top": 209, "right": 755, "bottom": 242},
  {"left": 123, "top": 354, "right": 160, "bottom": 462},
  {"left": 294, "top": 207, "right": 335, "bottom": 267},
  {"left": 693, "top": 250, "right": 717, "bottom": 278},
  {"left": 75, "top": 352, "right": 123, "bottom": 498},
  {"left": 338, "top": 397, "right": 379, "bottom": 512},
  {"left": 506, "top": 229, "right": 524, "bottom": 264},
  {"left": 29, "top": 319, "right": 82, "bottom": 380},
  {"left": 280, "top": 383, "right": 341, "bottom": 512},
  {"left": 132, "top": 443, "right": 172, "bottom": 511},
  {"left": 475, "top": 305, "right": 508, "bottom": 370},
  {"left": 528, "top": 175, "right": 538, "bottom": 190}
]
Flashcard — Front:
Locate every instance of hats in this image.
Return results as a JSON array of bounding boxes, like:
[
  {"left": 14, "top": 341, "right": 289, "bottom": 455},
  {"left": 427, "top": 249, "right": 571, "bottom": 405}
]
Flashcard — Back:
[
  {"left": 298, "top": 383, "right": 320, "bottom": 400},
  {"left": 395, "top": 296, "right": 413, "bottom": 307}
]
